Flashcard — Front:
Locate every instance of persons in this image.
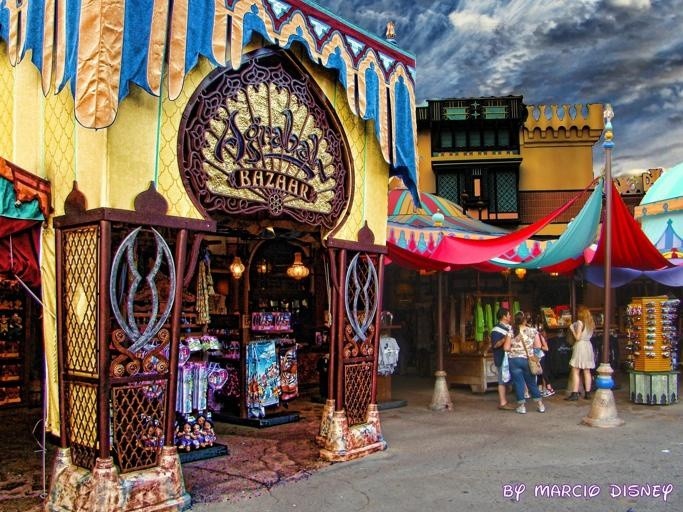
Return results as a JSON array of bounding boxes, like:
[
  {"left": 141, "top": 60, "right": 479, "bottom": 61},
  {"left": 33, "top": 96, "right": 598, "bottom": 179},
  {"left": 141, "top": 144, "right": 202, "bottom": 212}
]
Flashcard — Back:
[
  {"left": 491, "top": 308, "right": 555, "bottom": 414},
  {"left": 564, "top": 304, "right": 595, "bottom": 401}
]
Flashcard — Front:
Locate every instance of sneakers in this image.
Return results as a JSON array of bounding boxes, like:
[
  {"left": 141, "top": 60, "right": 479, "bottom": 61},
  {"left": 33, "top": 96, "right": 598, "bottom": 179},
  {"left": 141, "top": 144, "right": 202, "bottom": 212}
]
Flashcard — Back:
[
  {"left": 515, "top": 405, "right": 526, "bottom": 414},
  {"left": 497, "top": 400, "right": 515, "bottom": 410},
  {"left": 542, "top": 389, "right": 555, "bottom": 398},
  {"left": 537, "top": 405, "right": 546, "bottom": 412}
]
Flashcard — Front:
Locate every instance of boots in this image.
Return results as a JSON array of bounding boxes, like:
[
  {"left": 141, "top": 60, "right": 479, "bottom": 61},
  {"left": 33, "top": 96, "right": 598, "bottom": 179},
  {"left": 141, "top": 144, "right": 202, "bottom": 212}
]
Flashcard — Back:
[
  {"left": 563, "top": 393, "right": 578, "bottom": 401},
  {"left": 583, "top": 392, "right": 592, "bottom": 399}
]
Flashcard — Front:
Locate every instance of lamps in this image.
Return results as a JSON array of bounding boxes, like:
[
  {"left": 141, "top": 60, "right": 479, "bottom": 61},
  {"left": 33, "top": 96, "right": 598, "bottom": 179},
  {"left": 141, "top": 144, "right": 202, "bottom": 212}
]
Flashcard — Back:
[{"left": 228, "top": 236, "right": 310, "bottom": 282}]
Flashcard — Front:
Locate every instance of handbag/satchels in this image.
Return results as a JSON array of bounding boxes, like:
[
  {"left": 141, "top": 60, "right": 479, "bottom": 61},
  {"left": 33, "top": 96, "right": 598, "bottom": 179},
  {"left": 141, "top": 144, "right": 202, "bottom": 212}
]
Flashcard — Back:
[
  {"left": 528, "top": 356, "right": 544, "bottom": 376},
  {"left": 501, "top": 354, "right": 511, "bottom": 383}
]
